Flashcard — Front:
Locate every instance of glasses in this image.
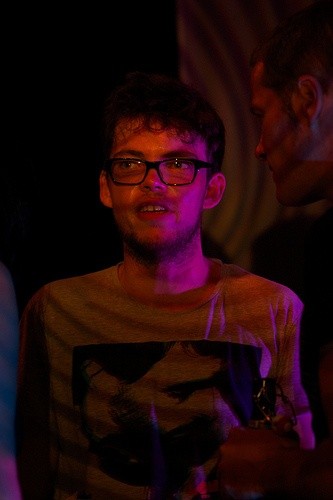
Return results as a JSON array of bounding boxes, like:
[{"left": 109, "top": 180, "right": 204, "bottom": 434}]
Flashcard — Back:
[{"left": 105, "top": 157, "right": 216, "bottom": 188}]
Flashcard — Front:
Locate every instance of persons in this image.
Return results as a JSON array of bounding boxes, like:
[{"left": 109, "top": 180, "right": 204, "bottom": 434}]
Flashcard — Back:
[
  {"left": 16, "top": 77, "right": 315, "bottom": 499},
  {"left": 245, "top": 5, "right": 333, "bottom": 445},
  {"left": 217, "top": 336, "right": 333, "bottom": 500},
  {"left": 0, "top": 259, "right": 22, "bottom": 500}
]
